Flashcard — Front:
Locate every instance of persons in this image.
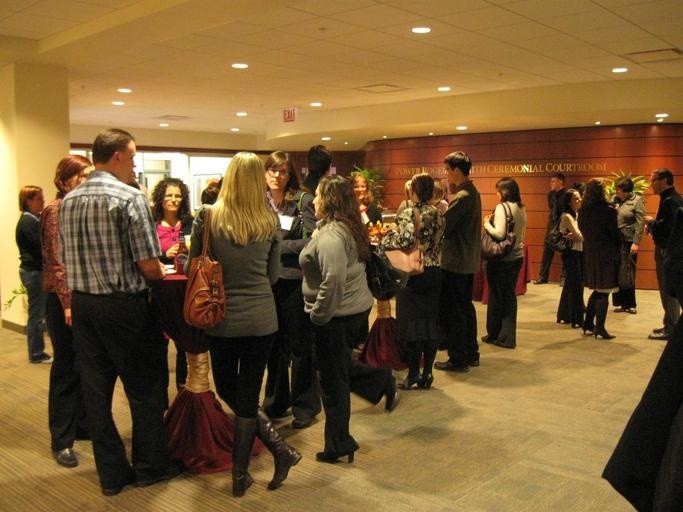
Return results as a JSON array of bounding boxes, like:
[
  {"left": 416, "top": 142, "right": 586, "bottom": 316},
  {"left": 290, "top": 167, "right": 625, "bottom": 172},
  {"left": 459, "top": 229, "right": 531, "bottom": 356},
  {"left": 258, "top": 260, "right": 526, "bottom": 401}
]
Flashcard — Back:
[
  {"left": 200, "top": 177, "right": 224, "bottom": 206},
  {"left": 14, "top": 185, "right": 57, "bottom": 365},
  {"left": 57, "top": 127, "right": 188, "bottom": 496},
  {"left": 533, "top": 173, "right": 567, "bottom": 286},
  {"left": 300, "top": 146, "right": 481, "bottom": 465},
  {"left": 150, "top": 176, "right": 193, "bottom": 418},
  {"left": 182, "top": 151, "right": 304, "bottom": 498},
  {"left": 642, "top": 167, "right": 683, "bottom": 339},
  {"left": 481, "top": 177, "right": 528, "bottom": 349},
  {"left": 37, "top": 153, "right": 93, "bottom": 468},
  {"left": 259, "top": 150, "right": 322, "bottom": 430},
  {"left": 556, "top": 176, "right": 645, "bottom": 339}
]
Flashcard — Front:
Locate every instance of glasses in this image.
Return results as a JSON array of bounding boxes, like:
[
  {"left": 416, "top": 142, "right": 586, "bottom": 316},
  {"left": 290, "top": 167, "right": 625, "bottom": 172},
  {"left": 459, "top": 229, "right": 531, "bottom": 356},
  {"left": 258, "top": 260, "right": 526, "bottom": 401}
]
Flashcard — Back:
[
  {"left": 267, "top": 168, "right": 289, "bottom": 177},
  {"left": 163, "top": 195, "right": 182, "bottom": 201},
  {"left": 651, "top": 178, "right": 664, "bottom": 181}
]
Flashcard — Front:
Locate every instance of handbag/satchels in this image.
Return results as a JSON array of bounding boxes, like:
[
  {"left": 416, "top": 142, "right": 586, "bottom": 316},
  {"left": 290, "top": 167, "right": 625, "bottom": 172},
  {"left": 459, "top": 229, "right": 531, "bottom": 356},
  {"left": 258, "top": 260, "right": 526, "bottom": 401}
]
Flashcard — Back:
[
  {"left": 480, "top": 201, "right": 516, "bottom": 262},
  {"left": 364, "top": 246, "right": 409, "bottom": 302},
  {"left": 544, "top": 222, "right": 574, "bottom": 254},
  {"left": 183, "top": 256, "right": 228, "bottom": 330},
  {"left": 384, "top": 205, "right": 425, "bottom": 277}
]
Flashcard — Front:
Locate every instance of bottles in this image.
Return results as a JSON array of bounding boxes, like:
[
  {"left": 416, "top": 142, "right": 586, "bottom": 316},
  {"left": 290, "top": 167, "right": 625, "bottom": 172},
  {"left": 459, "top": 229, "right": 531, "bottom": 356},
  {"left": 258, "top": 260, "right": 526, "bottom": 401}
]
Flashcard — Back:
[{"left": 174, "top": 230, "right": 190, "bottom": 275}]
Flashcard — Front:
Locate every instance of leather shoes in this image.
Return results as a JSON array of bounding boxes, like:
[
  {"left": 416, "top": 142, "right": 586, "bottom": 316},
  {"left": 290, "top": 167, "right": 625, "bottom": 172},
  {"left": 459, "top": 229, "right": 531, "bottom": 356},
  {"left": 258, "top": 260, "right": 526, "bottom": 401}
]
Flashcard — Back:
[
  {"left": 433, "top": 363, "right": 469, "bottom": 373},
  {"left": 50, "top": 447, "right": 78, "bottom": 467},
  {"left": 469, "top": 353, "right": 480, "bottom": 367},
  {"left": 292, "top": 417, "right": 314, "bottom": 429}
]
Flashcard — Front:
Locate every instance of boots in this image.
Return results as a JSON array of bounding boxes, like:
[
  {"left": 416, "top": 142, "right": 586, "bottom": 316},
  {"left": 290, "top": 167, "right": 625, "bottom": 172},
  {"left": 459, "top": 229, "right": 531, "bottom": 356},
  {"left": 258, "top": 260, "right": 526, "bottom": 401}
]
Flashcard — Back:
[
  {"left": 255, "top": 407, "right": 302, "bottom": 490},
  {"left": 232, "top": 417, "right": 257, "bottom": 496}
]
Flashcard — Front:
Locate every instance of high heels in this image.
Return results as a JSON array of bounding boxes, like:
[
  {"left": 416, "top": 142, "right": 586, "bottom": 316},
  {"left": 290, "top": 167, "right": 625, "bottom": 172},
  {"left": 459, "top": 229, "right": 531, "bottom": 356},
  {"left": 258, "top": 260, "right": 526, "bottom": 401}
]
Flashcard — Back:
[
  {"left": 556, "top": 315, "right": 571, "bottom": 323},
  {"left": 384, "top": 375, "right": 402, "bottom": 413},
  {"left": 581, "top": 323, "right": 596, "bottom": 335},
  {"left": 316, "top": 450, "right": 355, "bottom": 464},
  {"left": 593, "top": 328, "right": 616, "bottom": 339},
  {"left": 572, "top": 320, "right": 585, "bottom": 328},
  {"left": 420, "top": 374, "right": 433, "bottom": 390},
  {"left": 397, "top": 374, "right": 422, "bottom": 389}
]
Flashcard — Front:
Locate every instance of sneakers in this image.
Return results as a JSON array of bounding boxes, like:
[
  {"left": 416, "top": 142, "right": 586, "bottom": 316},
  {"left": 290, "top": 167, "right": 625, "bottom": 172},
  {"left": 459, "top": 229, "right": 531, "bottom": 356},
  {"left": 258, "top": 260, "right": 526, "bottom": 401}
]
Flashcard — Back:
[
  {"left": 101, "top": 464, "right": 134, "bottom": 496},
  {"left": 653, "top": 328, "right": 665, "bottom": 333},
  {"left": 560, "top": 280, "right": 565, "bottom": 287},
  {"left": 648, "top": 333, "right": 670, "bottom": 340},
  {"left": 533, "top": 279, "right": 549, "bottom": 284},
  {"left": 132, "top": 464, "right": 181, "bottom": 487},
  {"left": 29, "top": 354, "right": 54, "bottom": 363},
  {"left": 628, "top": 307, "right": 636, "bottom": 314},
  {"left": 614, "top": 306, "right": 629, "bottom": 312}
]
